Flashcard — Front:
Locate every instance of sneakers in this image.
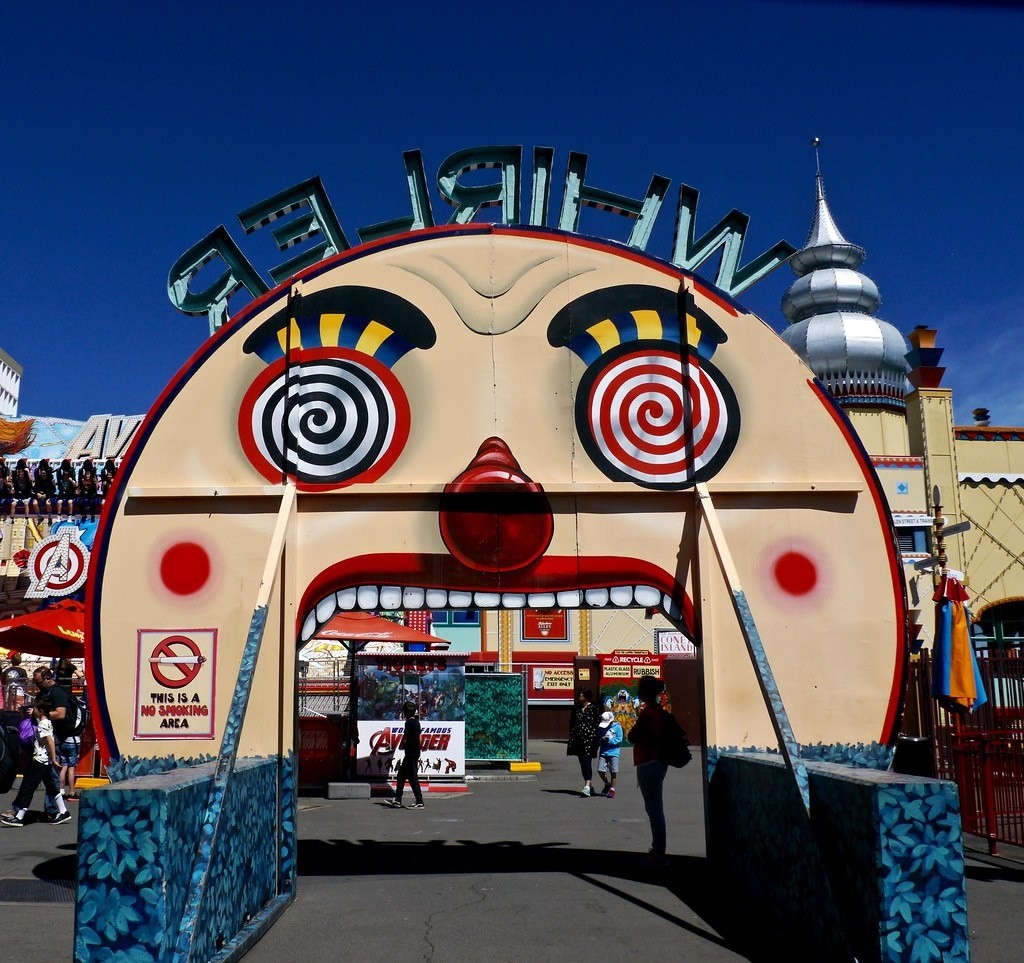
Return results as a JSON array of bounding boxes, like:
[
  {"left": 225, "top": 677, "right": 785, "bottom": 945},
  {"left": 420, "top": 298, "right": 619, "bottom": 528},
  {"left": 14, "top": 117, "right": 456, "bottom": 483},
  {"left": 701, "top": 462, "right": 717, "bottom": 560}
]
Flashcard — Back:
[
  {"left": 601, "top": 783, "right": 611, "bottom": 796},
  {"left": 607, "top": 790, "right": 616, "bottom": 798},
  {"left": 406, "top": 803, "right": 425, "bottom": 810},
  {"left": 384, "top": 797, "right": 401, "bottom": 807},
  {"left": 581, "top": 786, "right": 596, "bottom": 797}
]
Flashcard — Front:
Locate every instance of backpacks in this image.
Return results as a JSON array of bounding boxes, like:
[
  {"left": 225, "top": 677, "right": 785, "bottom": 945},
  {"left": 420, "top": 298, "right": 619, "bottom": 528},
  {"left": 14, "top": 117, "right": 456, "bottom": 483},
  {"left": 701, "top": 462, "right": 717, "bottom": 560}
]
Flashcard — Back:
[{"left": 660, "top": 710, "right": 692, "bottom": 769}]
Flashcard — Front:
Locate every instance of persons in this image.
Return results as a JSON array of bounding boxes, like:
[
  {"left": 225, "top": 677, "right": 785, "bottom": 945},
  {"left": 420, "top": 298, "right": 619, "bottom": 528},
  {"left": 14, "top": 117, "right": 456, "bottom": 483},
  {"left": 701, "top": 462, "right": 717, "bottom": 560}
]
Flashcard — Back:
[
  {"left": 384, "top": 701, "right": 425, "bottom": 810},
  {"left": 0, "top": 650, "right": 86, "bottom": 828},
  {"left": 567, "top": 689, "right": 605, "bottom": 797},
  {"left": 0, "top": 459, "right": 117, "bottom": 525},
  {"left": 598, "top": 711, "right": 624, "bottom": 799},
  {"left": 626, "top": 676, "right": 692, "bottom": 856}
]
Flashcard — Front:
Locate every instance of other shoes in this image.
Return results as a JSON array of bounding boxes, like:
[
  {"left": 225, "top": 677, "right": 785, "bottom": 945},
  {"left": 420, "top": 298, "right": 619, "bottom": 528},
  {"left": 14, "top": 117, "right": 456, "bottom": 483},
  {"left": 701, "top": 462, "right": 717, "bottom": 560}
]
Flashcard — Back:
[
  {"left": 63, "top": 793, "right": 80, "bottom": 802},
  {"left": 50, "top": 811, "right": 71, "bottom": 825},
  {"left": 0, "top": 810, "right": 17, "bottom": 818},
  {"left": 36, "top": 813, "right": 56, "bottom": 822},
  {"left": 1, "top": 816, "right": 24, "bottom": 827}
]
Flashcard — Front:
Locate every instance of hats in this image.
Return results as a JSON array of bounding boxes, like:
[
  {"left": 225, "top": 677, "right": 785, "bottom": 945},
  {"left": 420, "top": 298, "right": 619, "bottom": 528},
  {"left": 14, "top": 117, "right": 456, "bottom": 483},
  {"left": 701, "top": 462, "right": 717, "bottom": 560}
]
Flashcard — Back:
[{"left": 598, "top": 711, "right": 615, "bottom": 728}]
